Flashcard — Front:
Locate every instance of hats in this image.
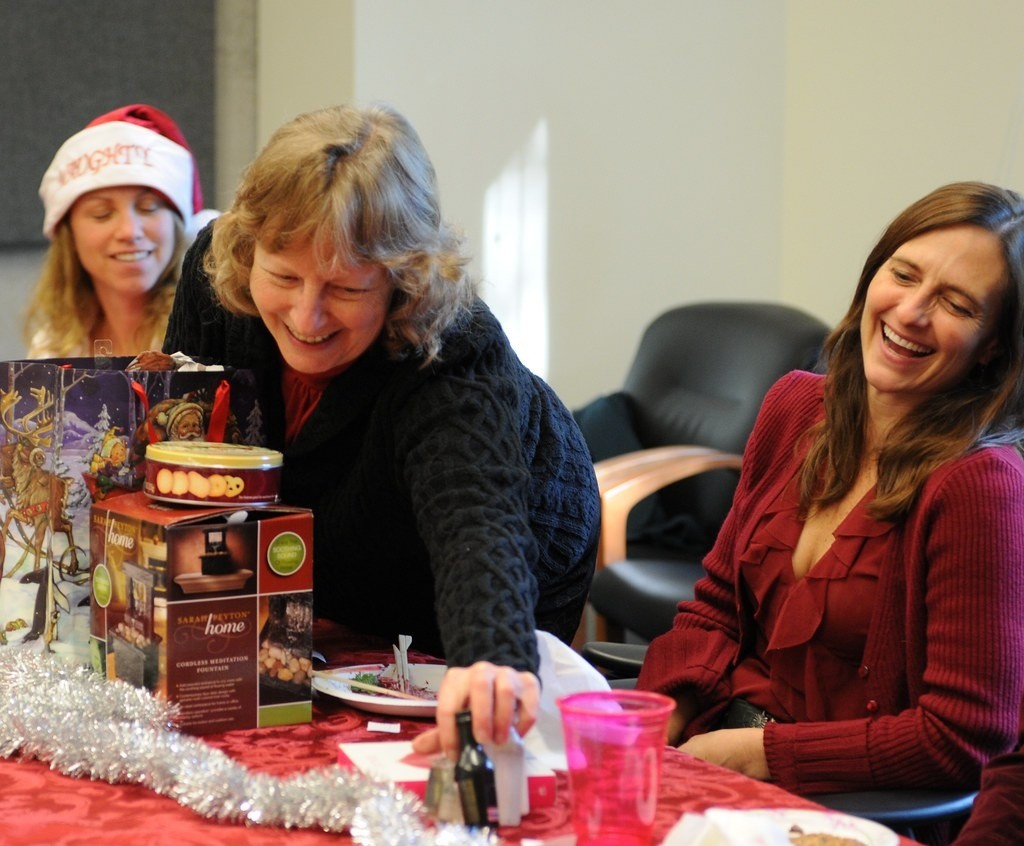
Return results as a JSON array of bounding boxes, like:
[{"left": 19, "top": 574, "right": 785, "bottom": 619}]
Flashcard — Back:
[{"left": 39, "top": 103, "right": 202, "bottom": 242}]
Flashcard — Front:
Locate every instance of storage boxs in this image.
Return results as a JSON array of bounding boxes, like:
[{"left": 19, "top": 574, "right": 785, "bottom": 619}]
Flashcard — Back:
[{"left": 5, "top": 343, "right": 318, "bottom": 730}]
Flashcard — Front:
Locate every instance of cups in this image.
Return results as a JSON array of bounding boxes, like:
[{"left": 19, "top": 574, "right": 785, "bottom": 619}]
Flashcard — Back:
[{"left": 558, "top": 688, "right": 675, "bottom": 846}]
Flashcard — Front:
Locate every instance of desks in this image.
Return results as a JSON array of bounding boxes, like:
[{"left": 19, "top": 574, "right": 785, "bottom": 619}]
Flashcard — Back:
[{"left": 1, "top": 611, "right": 931, "bottom": 844}]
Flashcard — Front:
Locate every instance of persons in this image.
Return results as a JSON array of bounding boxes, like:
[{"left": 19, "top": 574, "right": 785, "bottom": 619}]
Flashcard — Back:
[
  {"left": 634, "top": 181, "right": 1024, "bottom": 795},
  {"left": 160, "top": 102, "right": 601, "bottom": 763},
  {"left": 17, "top": 103, "right": 204, "bottom": 360}
]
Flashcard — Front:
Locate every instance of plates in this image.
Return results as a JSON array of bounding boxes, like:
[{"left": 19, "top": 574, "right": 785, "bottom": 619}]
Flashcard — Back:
[
  {"left": 705, "top": 805, "right": 903, "bottom": 845},
  {"left": 310, "top": 659, "right": 471, "bottom": 719}
]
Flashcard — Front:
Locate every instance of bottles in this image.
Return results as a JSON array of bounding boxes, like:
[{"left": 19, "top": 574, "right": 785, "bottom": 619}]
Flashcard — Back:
[
  {"left": 422, "top": 751, "right": 458, "bottom": 826},
  {"left": 491, "top": 715, "right": 524, "bottom": 828},
  {"left": 454, "top": 712, "right": 499, "bottom": 831}
]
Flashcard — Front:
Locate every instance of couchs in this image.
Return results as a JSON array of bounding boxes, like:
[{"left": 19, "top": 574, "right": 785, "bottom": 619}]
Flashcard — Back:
[{"left": 568, "top": 297, "right": 836, "bottom": 645}]
